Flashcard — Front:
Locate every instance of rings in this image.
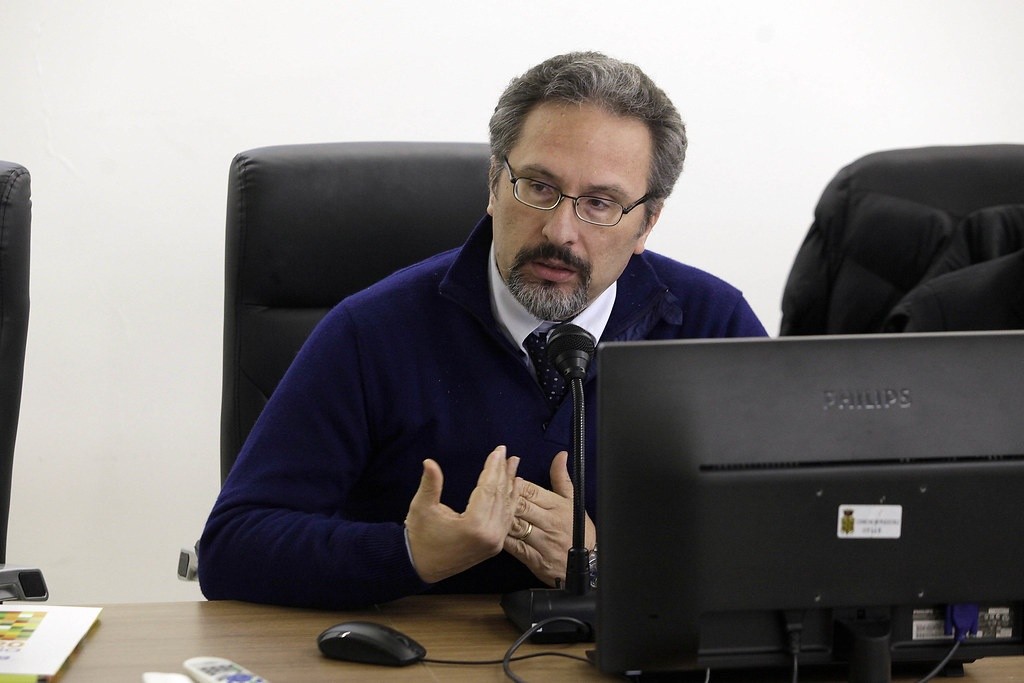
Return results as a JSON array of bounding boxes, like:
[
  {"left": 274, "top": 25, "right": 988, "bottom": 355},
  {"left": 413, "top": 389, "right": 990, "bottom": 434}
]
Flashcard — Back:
[{"left": 521, "top": 523, "right": 532, "bottom": 540}]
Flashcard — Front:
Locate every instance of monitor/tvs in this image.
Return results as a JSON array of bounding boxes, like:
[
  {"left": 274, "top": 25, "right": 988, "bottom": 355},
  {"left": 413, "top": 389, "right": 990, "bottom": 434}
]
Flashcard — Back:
[{"left": 585, "top": 327, "right": 1024, "bottom": 682}]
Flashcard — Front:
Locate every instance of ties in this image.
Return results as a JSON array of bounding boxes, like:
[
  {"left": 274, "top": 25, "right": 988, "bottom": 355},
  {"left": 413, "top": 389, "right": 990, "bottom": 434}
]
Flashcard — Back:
[{"left": 523, "top": 333, "right": 571, "bottom": 406}]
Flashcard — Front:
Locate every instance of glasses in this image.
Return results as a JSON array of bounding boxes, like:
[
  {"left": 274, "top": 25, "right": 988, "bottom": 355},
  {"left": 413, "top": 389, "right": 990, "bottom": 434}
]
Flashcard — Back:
[{"left": 500, "top": 152, "right": 659, "bottom": 226}]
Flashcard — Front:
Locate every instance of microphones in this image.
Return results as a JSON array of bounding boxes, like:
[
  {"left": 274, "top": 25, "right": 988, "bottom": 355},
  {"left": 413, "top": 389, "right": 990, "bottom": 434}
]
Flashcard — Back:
[{"left": 500, "top": 322, "right": 597, "bottom": 643}]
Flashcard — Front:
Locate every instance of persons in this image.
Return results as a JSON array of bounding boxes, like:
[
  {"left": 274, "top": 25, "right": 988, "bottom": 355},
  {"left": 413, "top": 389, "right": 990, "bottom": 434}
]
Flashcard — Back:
[{"left": 198, "top": 53, "right": 773, "bottom": 613}]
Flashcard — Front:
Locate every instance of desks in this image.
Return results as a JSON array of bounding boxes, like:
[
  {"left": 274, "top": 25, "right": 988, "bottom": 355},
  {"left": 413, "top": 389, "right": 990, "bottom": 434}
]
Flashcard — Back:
[{"left": 0, "top": 598, "right": 1024, "bottom": 683}]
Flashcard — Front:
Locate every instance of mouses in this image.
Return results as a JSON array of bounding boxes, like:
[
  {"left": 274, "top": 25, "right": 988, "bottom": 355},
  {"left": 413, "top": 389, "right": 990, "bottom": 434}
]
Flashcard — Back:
[{"left": 317, "top": 621, "right": 426, "bottom": 666}]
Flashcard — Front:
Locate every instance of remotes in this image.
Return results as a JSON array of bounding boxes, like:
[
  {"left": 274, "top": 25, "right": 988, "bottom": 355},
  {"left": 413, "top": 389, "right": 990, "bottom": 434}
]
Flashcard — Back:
[{"left": 185, "top": 656, "right": 270, "bottom": 682}]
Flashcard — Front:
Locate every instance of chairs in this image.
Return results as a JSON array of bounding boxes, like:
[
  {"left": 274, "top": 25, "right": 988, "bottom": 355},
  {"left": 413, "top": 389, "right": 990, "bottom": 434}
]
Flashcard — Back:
[
  {"left": 219, "top": 141, "right": 491, "bottom": 504},
  {"left": 775, "top": 144, "right": 1024, "bottom": 338}
]
